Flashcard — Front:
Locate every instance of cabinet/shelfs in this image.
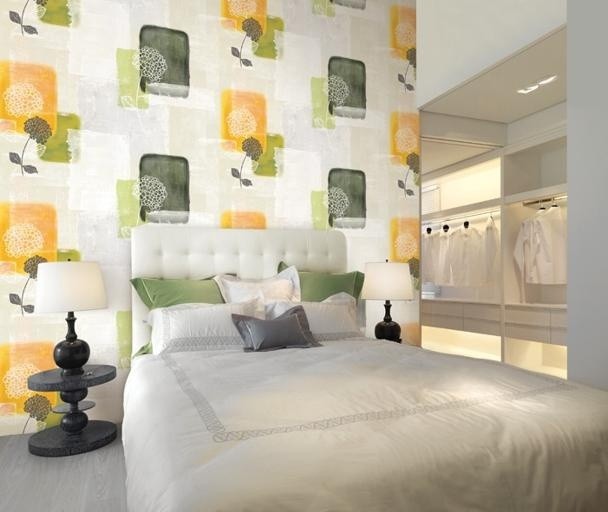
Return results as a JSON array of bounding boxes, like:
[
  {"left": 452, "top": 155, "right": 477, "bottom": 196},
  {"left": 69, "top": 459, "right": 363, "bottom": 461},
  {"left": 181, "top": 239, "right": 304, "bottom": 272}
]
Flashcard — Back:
[
  {"left": 421, "top": 152, "right": 499, "bottom": 337},
  {"left": 501, "top": 124, "right": 568, "bottom": 347}
]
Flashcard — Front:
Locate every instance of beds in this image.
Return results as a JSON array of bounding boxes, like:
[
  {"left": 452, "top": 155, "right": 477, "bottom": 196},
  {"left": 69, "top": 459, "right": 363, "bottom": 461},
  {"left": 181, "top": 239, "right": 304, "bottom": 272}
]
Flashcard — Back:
[{"left": 123, "top": 227, "right": 607, "bottom": 512}]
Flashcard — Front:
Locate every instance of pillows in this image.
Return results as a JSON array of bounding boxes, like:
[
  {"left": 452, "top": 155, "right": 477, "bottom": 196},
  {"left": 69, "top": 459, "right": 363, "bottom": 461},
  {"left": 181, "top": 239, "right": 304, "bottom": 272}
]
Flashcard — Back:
[{"left": 128, "top": 260, "right": 365, "bottom": 357}]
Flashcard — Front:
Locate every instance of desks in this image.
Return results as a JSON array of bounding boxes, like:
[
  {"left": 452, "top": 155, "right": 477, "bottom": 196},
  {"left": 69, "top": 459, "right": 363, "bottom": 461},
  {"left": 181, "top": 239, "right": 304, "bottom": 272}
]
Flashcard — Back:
[{"left": 27, "top": 364, "right": 118, "bottom": 459}]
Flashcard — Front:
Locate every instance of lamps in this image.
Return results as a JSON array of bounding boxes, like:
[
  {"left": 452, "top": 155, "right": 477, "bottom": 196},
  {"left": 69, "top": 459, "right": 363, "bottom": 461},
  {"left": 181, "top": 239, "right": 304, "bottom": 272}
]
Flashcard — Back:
[
  {"left": 359, "top": 260, "right": 416, "bottom": 343},
  {"left": 30, "top": 259, "right": 112, "bottom": 378}
]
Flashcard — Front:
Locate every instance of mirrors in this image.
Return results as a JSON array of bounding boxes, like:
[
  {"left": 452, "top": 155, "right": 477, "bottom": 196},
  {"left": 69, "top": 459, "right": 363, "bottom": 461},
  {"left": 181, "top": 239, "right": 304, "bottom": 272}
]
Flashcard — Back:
[{"left": 419, "top": 136, "right": 500, "bottom": 364}]
